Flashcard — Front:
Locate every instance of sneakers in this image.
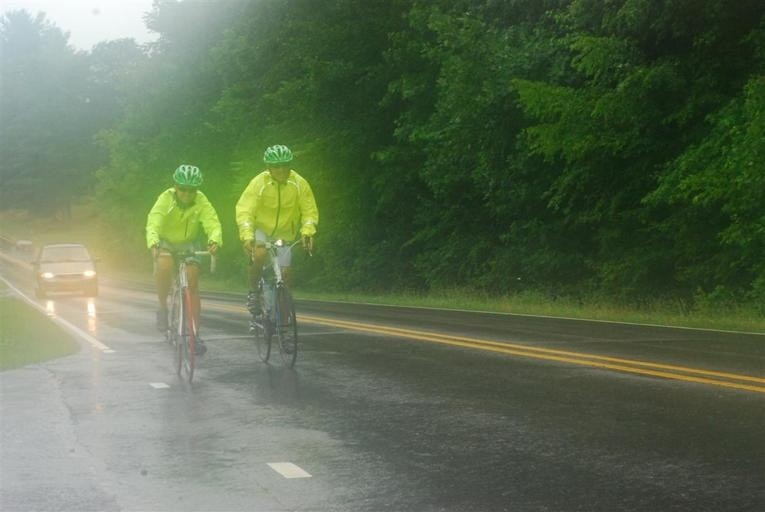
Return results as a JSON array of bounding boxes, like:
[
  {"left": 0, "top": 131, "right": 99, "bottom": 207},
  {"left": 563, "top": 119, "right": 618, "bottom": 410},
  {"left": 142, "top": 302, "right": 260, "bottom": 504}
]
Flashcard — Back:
[
  {"left": 280, "top": 331, "right": 294, "bottom": 354},
  {"left": 157, "top": 310, "right": 169, "bottom": 329},
  {"left": 195, "top": 340, "right": 207, "bottom": 355},
  {"left": 247, "top": 291, "right": 262, "bottom": 316}
]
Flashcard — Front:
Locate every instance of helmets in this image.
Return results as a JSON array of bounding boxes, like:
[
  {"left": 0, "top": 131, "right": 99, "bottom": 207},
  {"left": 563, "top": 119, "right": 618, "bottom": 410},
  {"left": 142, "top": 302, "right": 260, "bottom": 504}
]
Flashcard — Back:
[
  {"left": 173, "top": 164, "right": 203, "bottom": 186},
  {"left": 263, "top": 144, "right": 293, "bottom": 163}
]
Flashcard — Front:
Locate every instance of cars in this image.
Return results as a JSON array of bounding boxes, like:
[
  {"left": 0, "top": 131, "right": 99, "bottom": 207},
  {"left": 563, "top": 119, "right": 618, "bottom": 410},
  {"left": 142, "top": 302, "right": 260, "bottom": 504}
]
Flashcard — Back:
[{"left": 34, "top": 244, "right": 101, "bottom": 299}]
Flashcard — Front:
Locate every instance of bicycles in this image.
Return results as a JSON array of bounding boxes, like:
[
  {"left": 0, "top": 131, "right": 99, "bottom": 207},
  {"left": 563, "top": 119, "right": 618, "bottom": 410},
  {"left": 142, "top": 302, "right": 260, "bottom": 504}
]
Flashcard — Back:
[
  {"left": 248, "top": 239, "right": 311, "bottom": 369},
  {"left": 154, "top": 250, "right": 216, "bottom": 385}
]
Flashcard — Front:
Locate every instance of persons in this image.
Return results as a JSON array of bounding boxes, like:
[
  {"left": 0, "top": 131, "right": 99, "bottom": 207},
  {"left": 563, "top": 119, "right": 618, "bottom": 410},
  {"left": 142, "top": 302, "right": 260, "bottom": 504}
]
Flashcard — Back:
[
  {"left": 234, "top": 142, "right": 320, "bottom": 352},
  {"left": 144, "top": 164, "right": 223, "bottom": 355}
]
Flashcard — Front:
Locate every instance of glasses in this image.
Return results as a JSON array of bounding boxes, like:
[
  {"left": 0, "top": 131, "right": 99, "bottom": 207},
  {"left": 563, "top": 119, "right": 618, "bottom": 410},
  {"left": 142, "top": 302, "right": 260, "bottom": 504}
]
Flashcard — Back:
[{"left": 179, "top": 186, "right": 197, "bottom": 193}]
